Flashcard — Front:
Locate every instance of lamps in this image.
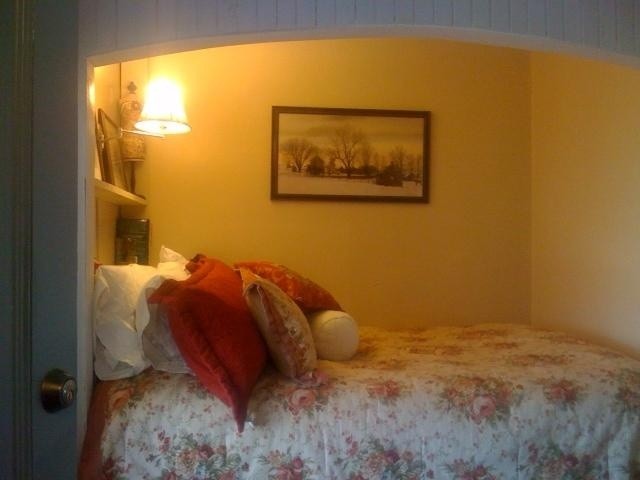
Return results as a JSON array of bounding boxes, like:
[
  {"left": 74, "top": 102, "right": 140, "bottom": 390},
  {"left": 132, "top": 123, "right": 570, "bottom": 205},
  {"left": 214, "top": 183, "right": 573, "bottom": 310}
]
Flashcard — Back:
[{"left": 98, "top": 79, "right": 192, "bottom": 156}]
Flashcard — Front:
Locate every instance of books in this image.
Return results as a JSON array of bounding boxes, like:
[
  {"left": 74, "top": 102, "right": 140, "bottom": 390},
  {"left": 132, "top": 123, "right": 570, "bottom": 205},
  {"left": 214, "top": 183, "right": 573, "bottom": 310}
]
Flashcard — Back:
[{"left": 114, "top": 218, "right": 150, "bottom": 266}]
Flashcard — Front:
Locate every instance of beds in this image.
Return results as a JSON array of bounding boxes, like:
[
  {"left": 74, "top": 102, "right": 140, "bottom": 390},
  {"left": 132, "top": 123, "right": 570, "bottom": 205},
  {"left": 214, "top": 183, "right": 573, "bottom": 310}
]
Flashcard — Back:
[{"left": 90, "top": 323, "right": 639, "bottom": 480}]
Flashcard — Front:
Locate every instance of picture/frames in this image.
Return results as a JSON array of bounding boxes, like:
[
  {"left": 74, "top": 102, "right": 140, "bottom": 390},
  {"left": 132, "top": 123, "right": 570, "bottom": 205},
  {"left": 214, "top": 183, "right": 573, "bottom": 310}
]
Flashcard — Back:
[
  {"left": 269, "top": 105, "right": 432, "bottom": 205},
  {"left": 97, "top": 106, "right": 131, "bottom": 193}
]
